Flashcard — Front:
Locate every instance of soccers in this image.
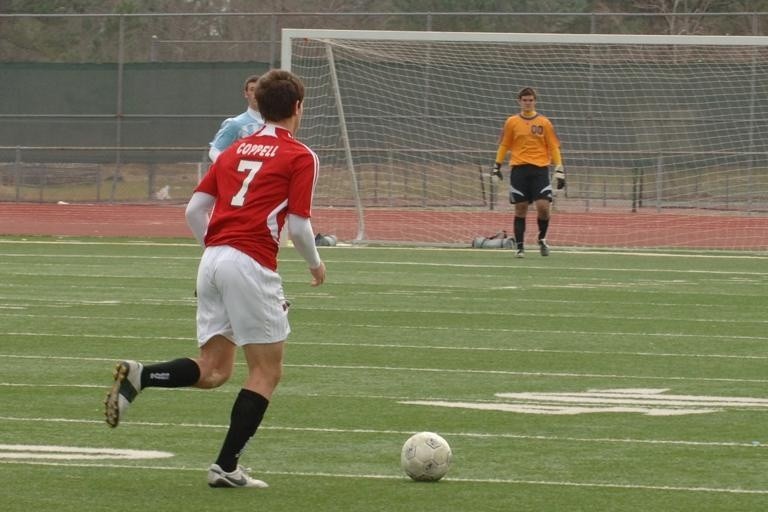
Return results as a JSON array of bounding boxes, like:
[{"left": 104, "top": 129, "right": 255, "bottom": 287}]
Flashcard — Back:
[{"left": 401, "top": 432, "right": 450, "bottom": 482}]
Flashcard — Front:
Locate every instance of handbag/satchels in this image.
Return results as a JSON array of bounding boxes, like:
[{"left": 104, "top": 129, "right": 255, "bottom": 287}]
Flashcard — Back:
[
  {"left": 471, "top": 230, "right": 516, "bottom": 250},
  {"left": 315, "top": 230, "right": 339, "bottom": 246}
]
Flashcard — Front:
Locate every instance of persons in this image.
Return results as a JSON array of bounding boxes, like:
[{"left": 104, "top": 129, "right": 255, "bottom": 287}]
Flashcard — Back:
[
  {"left": 491, "top": 87, "right": 565, "bottom": 257},
  {"left": 104, "top": 70, "right": 326, "bottom": 486},
  {"left": 208, "top": 73, "right": 265, "bottom": 168}
]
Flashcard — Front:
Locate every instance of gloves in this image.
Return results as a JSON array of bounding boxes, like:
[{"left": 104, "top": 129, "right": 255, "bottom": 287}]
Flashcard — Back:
[
  {"left": 492, "top": 162, "right": 504, "bottom": 183},
  {"left": 550, "top": 167, "right": 568, "bottom": 191}
]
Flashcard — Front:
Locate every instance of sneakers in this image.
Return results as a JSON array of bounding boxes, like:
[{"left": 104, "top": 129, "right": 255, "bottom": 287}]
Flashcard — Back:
[
  {"left": 103, "top": 358, "right": 143, "bottom": 428},
  {"left": 539, "top": 244, "right": 551, "bottom": 257},
  {"left": 514, "top": 248, "right": 526, "bottom": 258},
  {"left": 206, "top": 462, "right": 270, "bottom": 491}
]
